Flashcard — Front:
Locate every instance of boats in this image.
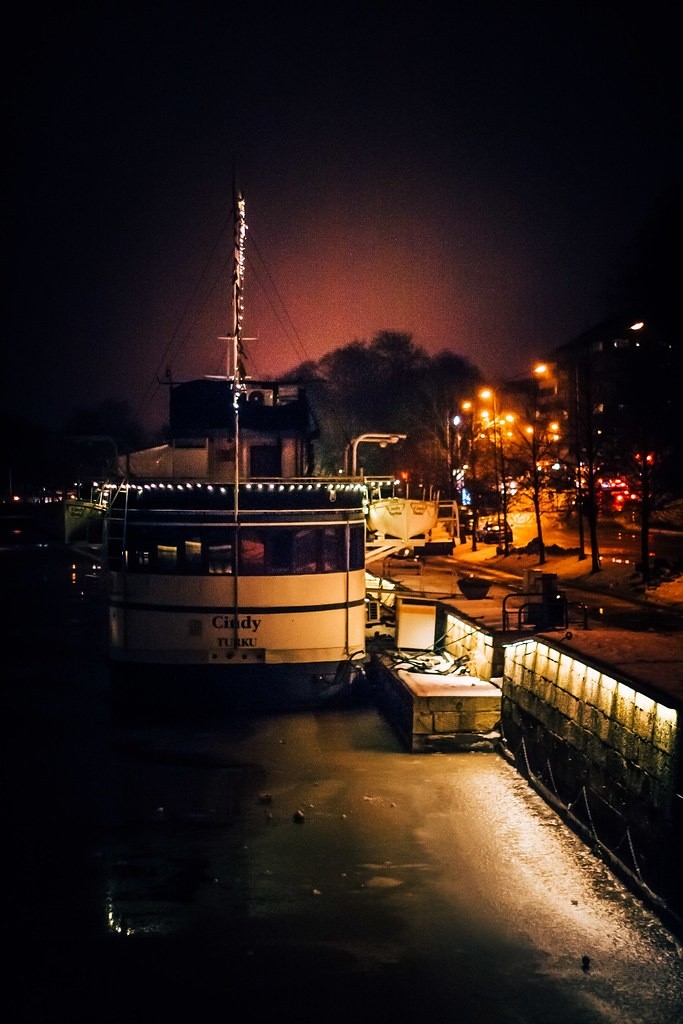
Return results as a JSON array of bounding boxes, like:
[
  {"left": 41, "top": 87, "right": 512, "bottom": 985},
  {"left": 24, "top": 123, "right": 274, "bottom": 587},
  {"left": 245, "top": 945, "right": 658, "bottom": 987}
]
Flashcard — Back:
[
  {"left": 54, "top": 183, "right": 377, "bottom": 701},
  {"left": 363, "top": 472, "right": 439, "bottom": 541}
]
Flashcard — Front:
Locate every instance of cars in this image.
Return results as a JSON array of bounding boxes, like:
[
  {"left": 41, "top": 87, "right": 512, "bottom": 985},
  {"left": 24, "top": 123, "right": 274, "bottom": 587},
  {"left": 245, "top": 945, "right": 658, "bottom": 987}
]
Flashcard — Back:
[{"left": 479, "top": 519, "right": 515, "bottom": 544}]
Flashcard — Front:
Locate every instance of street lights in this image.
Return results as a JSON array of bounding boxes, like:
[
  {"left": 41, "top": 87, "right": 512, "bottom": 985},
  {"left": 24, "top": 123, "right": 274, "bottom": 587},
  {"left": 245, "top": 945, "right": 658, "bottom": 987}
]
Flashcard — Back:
[
  {"left": 462, "top": 400, "right": 480, "bottom": 551},
  {"left": 533, "top": 365, "right": 601, "bottom": 572},
  {"left": 480, "top": 389, "right": 511, "bottom": 557}
]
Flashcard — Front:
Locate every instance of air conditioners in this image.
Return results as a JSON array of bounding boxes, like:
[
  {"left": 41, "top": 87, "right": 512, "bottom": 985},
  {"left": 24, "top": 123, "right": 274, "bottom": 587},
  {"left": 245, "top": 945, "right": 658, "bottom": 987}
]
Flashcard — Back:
[
  {"left": 246, "top": 389, "right": 273, "bottom": 406},
  {"left": 368, "top": 601, "right": 380, "bottom": 621}
]
